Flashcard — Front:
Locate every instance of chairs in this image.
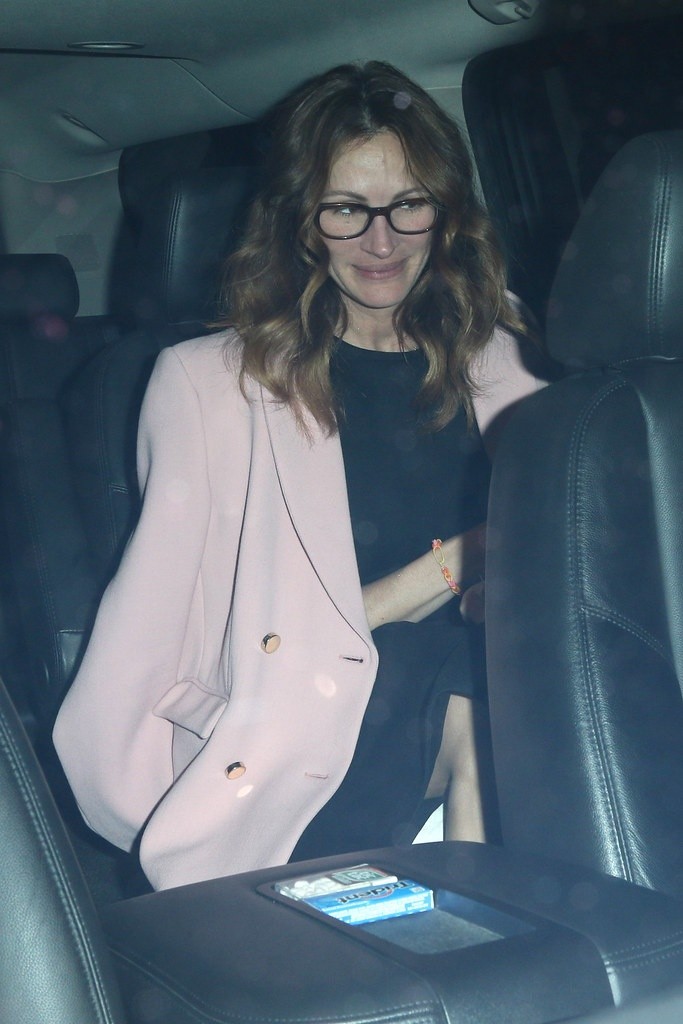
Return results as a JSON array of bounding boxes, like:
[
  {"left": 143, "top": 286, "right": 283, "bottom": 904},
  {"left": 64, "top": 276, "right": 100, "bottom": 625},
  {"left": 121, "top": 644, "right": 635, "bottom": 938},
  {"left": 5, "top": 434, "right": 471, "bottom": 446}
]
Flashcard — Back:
[{"left": 2, "top": 127, "right": 683, "bottom": 1024}]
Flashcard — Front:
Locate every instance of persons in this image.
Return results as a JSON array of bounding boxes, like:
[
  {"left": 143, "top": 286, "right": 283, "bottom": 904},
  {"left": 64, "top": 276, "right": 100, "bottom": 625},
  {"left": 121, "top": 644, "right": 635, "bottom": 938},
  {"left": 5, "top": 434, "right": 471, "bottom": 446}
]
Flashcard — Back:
[{"left": 53, "top": 60, "right": 551, "bottom": 893}]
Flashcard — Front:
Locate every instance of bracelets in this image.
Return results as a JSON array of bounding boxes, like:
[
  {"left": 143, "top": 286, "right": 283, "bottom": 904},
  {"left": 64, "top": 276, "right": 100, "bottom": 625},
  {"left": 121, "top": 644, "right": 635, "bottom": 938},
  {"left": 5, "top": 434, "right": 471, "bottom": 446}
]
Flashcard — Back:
[{"left": 432, "top": 539, "right": 462, "bottom": 595}]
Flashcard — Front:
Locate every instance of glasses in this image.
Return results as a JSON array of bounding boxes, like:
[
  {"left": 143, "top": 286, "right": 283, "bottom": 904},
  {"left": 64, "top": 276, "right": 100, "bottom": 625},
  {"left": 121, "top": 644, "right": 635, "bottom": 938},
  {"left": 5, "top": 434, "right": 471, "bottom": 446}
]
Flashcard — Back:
[{"left": 314, "top": 195, "right": 439, "bottom": 239}]
